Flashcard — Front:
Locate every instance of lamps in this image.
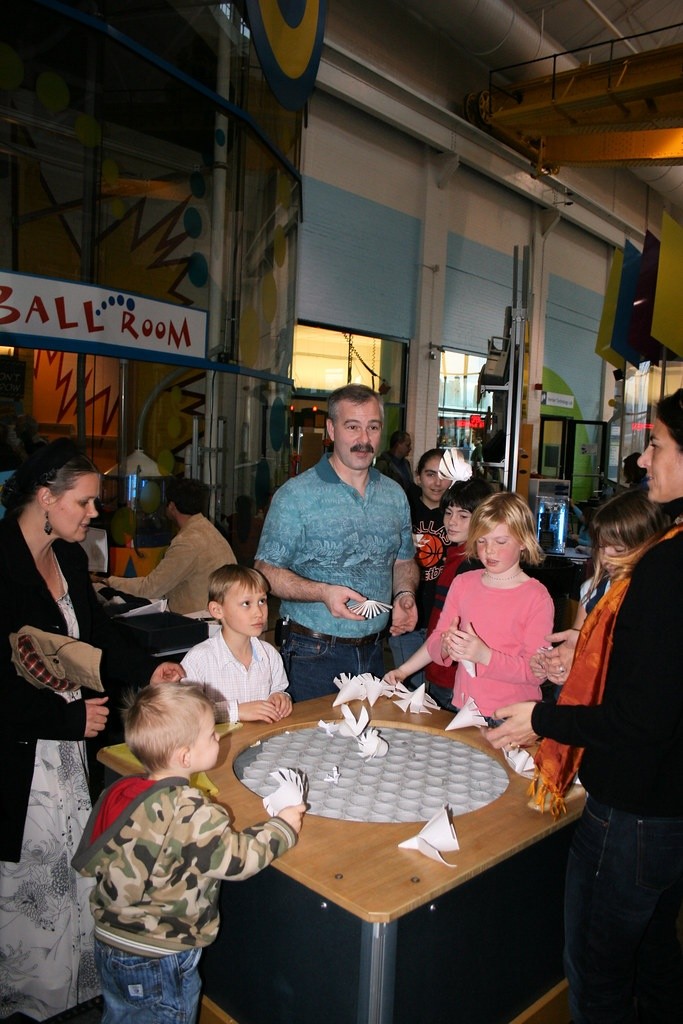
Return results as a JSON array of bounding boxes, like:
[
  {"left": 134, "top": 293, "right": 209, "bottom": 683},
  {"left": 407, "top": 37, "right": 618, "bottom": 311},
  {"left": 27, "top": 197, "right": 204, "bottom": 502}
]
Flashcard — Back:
[
  {"left": 429, "top": 340, "right": 445, "bottom": 353},
  {"left": 102, "top": 367, "right": 188, "bottom": 477}
]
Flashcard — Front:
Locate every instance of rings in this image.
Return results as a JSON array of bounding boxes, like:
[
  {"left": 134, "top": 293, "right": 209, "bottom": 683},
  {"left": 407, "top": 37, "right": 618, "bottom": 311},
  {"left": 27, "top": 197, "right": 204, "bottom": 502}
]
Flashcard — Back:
[
  {"left": 510, "top": 742, "right": 515, "bottom": 750},
  {"left": 560, "top": 666, "right": 564, "bottom": 673}
]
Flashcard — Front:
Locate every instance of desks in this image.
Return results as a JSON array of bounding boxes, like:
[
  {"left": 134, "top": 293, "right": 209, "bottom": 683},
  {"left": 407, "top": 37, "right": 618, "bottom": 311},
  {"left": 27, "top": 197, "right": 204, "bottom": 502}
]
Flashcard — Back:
[{"left": 96, "top": 690, "right": 586, "bottom": 1024}]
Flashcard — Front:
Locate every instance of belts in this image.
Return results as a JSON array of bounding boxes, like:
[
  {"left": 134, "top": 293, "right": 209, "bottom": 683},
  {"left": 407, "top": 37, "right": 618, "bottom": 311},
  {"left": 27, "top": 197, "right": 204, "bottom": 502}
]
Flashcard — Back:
[{"left": 289, "top": 620, "right": 390, "bottom": 645}]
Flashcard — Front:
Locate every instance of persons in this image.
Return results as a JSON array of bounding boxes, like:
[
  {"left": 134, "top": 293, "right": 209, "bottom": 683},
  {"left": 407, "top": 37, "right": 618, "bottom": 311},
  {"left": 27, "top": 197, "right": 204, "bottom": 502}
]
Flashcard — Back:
[
  {"left": 478, "top": 387, "right": 682, "bottom": 1024},
  {"left": 73, "top": 680, "right": 306, "bottom": 1024},
  {"left": 0, "top": 437, "right": 186, "bottom": 1024},
  {"left": 91, "top": 386, "right": 683, "bottom": 725}
]
